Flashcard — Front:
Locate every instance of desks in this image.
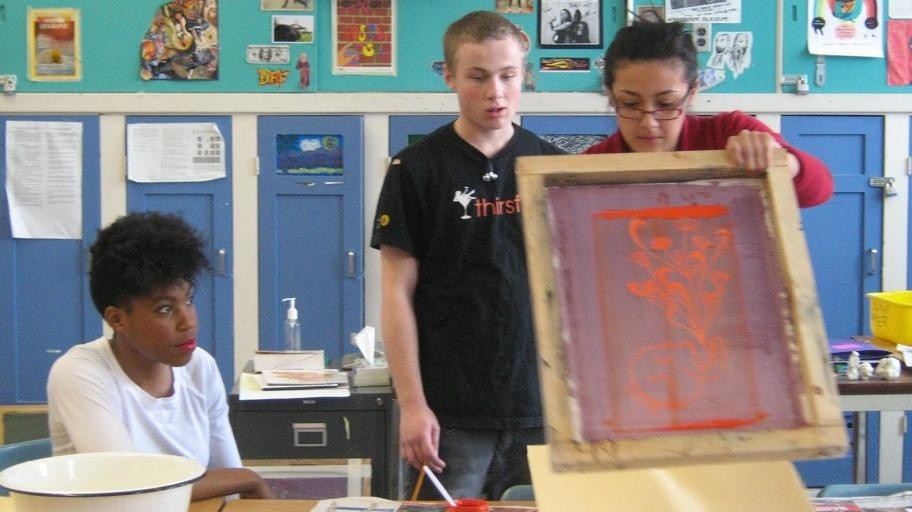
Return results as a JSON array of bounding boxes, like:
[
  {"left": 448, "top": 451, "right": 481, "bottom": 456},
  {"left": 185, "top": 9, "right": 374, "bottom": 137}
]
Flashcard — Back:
[{"left": 388, "top": 355, "right": 912, "bottom": 482}]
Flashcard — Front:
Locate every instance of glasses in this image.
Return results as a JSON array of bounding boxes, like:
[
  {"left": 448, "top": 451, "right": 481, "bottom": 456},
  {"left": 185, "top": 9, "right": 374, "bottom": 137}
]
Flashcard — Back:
[{"left": 610, "top": 89, "right": 691, "bottom": 123}]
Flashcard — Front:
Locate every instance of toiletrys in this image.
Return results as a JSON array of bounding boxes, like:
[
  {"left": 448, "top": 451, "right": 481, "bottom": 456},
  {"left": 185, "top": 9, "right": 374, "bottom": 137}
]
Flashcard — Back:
[{"left": 280, "top": 296, "right": 301, "bottom": 351}]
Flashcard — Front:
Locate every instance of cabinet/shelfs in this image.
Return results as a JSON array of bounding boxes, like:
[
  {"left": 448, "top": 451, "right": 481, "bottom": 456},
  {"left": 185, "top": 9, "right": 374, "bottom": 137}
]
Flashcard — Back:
[{"left": 228, "top": 357, "right": 392, "bottom": 492}]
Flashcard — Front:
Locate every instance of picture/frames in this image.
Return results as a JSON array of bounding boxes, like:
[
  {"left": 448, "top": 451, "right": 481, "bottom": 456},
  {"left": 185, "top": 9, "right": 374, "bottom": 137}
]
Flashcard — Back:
[{"left": 513, "top": 146, "right": 851, "bottom": 473}]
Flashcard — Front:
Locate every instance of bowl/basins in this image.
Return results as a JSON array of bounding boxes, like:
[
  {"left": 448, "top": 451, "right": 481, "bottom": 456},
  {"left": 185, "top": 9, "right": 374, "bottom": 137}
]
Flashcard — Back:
[{"left": 0, "top": 452, "right": 208, "bottom": 512}]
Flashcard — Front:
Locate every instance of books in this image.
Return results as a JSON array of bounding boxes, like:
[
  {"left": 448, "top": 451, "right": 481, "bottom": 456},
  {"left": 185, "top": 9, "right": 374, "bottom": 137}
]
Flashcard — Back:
[{"left": 228, "top": 341, "right": 351, "bottom": 405}]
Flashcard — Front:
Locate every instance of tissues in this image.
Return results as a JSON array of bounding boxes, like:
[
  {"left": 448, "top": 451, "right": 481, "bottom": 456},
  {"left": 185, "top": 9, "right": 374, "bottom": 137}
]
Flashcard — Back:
[{"left": 350, "top": 326, "right": 391, "bottom": 386}]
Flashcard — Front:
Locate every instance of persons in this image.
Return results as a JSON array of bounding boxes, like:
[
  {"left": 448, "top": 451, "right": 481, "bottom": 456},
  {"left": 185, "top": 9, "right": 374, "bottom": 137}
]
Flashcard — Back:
[
  {"left": 710, "top": 31, "right": 732, "bottom": 68},
  {"left": 571, "top": 8, "right": 592, "bottom": 43},
  {"left": 546, "top": 7, "right": 573, "bottom": 43},
  {"left": 365, "top": 10, "right": 571, "bottom": 503},
  {"left": 579, "top": 19, "right": 836, "bottom": 210},
  {"left": 46, "top": 207, "right": 275, "bottom": 503},
  {"left": 730, "top": 31, "right": 749, "bottom": 71}
]
